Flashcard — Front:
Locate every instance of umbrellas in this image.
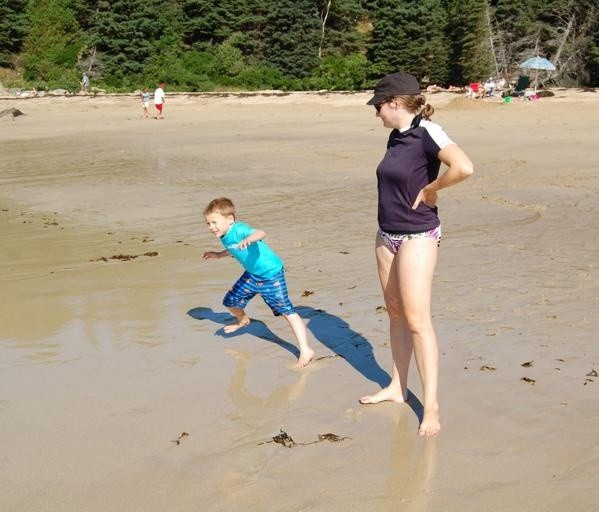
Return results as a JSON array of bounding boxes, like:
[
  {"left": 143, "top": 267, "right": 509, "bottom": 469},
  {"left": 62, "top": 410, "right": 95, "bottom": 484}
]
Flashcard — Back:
[{"left": 520, "top": 56, "right": 556, "bottom": 90}]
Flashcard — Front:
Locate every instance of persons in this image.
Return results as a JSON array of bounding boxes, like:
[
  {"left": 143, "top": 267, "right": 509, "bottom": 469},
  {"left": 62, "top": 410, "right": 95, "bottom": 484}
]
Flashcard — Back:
[
  {"left": 203, "top": 198, "right": 315, "bottom": 369},
  {"left": 140, "top": 87, "right": 152, "bottom": 119},
  {"left": 154, "top": 82, "right": 166, "bottom": 120},
  {"left": 468, "top": 75, "right": 507, "bottom": 100},
  {"left": 80, "top": 72, "right": 90, "bottom": 90},
  {"left": 357, "top": 72, "right": 474, "bottom": 436}
]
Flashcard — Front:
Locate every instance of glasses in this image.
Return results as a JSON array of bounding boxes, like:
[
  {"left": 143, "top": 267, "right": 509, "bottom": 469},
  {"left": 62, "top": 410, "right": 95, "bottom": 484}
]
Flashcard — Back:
[{"left": 373, "top": 100, "right": 391, "bottom": 113}]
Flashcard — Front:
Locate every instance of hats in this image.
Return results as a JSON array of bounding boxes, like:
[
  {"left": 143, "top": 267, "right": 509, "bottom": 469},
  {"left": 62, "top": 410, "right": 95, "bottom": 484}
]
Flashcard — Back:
[{"left": 366, "top": 71, "right": 422, "bottom": 106}]
